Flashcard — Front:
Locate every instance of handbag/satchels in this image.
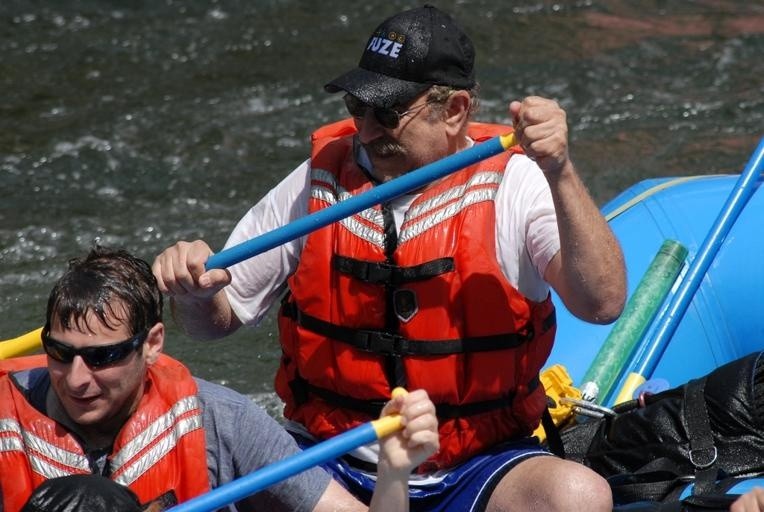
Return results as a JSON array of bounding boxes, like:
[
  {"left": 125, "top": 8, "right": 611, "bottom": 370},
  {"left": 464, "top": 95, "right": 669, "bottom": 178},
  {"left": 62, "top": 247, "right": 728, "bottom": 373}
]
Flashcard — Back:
[{"left": 581, "top": 349, "right": 764, "bottom": 503}]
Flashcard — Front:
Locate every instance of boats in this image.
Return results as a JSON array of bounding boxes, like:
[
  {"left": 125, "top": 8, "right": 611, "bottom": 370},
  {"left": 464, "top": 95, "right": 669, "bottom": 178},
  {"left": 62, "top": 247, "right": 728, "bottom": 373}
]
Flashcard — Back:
[{"left": 538, "top": 173, "right": 763, "bottom": 511}]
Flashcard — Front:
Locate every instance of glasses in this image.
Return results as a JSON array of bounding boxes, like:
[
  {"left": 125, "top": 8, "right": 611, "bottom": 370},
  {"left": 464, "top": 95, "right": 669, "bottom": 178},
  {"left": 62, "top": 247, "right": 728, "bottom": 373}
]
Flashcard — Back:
[
  {"left": 340, "top": 91, "right": 452, "bottom": 130},
  {"left": 40, "top": 321, "right": 149, "bottom": 369}
]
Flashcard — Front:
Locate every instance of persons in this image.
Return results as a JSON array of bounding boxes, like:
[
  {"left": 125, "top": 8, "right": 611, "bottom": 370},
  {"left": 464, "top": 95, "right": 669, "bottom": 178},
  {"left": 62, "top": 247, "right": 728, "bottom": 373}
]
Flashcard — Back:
[
  {"left": 731, "top": 486, "right": 764, "bottom": 512},
  {"left": 0, "top": 243, "right": 445, "bottom": 512},
  {"left": 150, "top": 5, "right": 626, "bottom": 512}
]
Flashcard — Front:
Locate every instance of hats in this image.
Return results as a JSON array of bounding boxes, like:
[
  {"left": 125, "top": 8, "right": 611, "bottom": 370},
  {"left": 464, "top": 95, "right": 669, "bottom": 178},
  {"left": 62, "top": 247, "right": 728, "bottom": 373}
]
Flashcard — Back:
[
  {"left": 18, "top": 474, "right": 144, "bottom": 512},
  {"left": 323, "top": 3, "right": 477, "bottom": 110}
]
